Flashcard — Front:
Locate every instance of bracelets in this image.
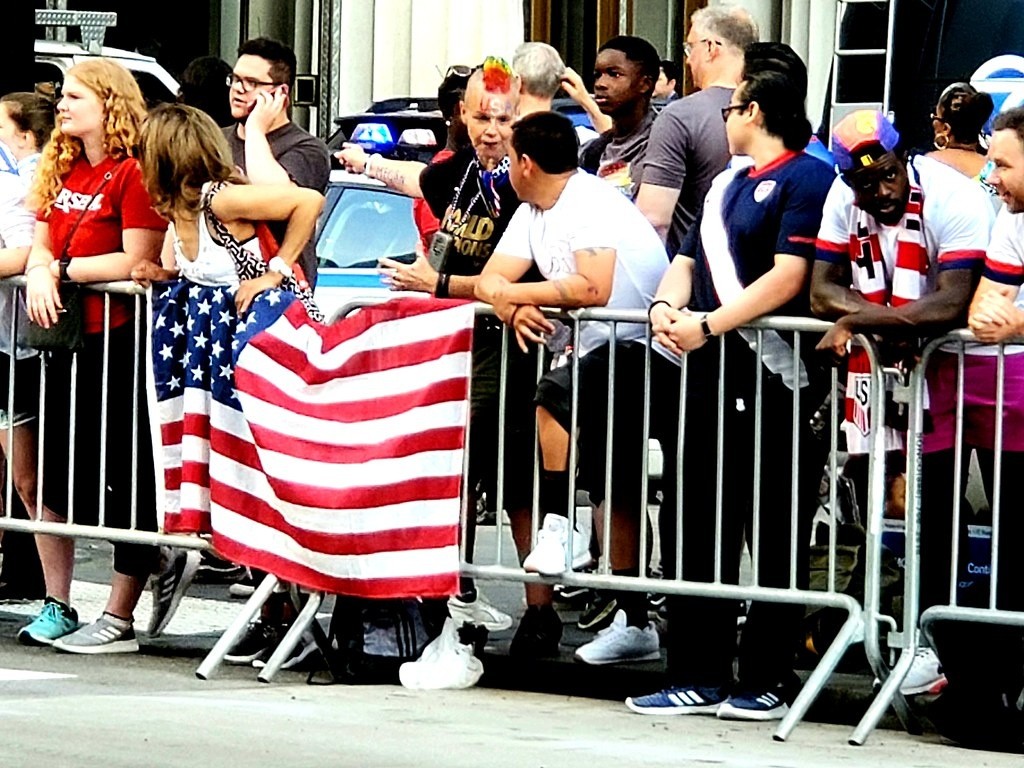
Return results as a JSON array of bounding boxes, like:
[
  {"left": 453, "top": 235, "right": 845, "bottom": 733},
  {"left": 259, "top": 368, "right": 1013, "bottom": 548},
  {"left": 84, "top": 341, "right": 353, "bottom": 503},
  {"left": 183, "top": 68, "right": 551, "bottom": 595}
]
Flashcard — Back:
[
  {"left": 700, "top": 314, "right": 716, "bottom": 341},
  {"left": 506, "top": 303, "right": 523, "bottom": 329},
  {"left": 435, "top": 271, "right": 450, "bottom": 297},
  {"left": 26, "top": 264, "right": 51, "bottom": 274},
  {"left": 649, "top": 300, "right": 671, "bottom": 317}
]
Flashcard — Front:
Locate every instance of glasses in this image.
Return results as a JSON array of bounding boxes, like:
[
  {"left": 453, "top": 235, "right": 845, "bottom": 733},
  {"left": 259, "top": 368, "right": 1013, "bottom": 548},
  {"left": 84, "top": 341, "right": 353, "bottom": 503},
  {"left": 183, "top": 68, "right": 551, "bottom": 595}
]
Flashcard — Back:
[
  {"left": 447, "top": 62, "right": 485, "bottom": 77},
  {"left": 721, "top": 103, "right": 749, "bottom": 122},
  {"left": 930, "top": 113, "right": 944, "bottom": 123},
  {"left": 226, "top": 73, "right": 282, "bottom": 91},
  {"left": 682, "top": 38, "right": 722, "bottom": 57}
]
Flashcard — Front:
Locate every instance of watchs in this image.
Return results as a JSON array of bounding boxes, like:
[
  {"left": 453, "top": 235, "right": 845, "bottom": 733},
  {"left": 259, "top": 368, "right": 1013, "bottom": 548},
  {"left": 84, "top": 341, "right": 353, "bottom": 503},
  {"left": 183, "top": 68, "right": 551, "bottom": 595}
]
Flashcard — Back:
[
  {"left": 267, "top": 256, "right": 292, "bottom": 279},
  {"left": 56, "top": 255, "right": 73, "bottom": 281}
]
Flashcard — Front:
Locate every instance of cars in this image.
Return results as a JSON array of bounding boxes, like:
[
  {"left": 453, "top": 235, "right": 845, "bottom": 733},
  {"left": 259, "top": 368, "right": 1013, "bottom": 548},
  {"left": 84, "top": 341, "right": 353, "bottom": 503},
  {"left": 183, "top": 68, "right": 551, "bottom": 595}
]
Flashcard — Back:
[{"left": 314, "top": 96, "right": 674, "bottom": 321}]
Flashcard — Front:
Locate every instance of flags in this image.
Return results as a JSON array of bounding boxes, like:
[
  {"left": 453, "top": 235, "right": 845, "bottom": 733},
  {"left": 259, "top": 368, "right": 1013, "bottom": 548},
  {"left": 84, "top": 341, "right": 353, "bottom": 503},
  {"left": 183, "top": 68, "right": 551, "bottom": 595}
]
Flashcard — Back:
[{"left": 144, "top": 282, "right": 474, "bottom": 596}]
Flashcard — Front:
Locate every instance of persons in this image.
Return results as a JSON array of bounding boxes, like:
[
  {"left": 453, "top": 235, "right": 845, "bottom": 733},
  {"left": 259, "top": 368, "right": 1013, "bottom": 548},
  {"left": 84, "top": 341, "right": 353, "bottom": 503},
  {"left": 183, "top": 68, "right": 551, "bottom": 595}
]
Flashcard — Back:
[{"left": 0, "top": 5, "right": 1024, "bottom": 719}]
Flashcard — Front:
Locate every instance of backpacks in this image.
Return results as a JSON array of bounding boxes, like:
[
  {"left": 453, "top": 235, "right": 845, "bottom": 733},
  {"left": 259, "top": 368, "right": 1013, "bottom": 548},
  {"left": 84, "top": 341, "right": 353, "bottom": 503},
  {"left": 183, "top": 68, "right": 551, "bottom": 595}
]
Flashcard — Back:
[{"left": 803, "top": 517, "right": 906, "bottom": 673}]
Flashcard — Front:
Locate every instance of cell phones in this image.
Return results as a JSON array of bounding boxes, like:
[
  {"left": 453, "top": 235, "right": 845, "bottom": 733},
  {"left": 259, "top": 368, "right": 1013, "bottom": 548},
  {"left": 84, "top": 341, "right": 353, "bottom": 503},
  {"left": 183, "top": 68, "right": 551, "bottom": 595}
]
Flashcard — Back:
[{"left": 248, "top": 84, "right": 290, "bottom": 114}]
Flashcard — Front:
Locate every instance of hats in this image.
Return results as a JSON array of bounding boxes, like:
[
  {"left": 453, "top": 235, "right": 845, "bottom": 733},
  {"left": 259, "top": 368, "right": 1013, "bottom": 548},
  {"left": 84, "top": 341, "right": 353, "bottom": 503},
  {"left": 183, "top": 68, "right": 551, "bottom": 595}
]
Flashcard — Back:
[{"left": 831, "top": 109, "right": 899, "bottom": 174}]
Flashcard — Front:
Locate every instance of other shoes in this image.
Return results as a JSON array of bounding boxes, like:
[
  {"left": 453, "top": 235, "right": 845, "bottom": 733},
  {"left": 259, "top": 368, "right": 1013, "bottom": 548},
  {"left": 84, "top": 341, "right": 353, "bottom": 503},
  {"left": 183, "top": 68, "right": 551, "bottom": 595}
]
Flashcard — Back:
[{"left": 191, "top": 550, "right": 260, "bottom": 596}]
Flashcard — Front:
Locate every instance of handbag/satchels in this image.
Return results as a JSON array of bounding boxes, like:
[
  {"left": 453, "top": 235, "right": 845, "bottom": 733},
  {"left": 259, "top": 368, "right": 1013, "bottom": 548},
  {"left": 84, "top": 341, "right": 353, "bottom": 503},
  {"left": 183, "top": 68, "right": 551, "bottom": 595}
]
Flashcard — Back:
[{"left": 18, "top": 283, "right": 86, "bottom": 350}]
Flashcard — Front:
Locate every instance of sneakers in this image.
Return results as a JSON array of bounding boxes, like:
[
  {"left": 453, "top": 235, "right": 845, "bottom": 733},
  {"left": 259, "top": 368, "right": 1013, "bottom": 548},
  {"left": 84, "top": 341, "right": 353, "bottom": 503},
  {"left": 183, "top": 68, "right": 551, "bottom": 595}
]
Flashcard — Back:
[
  {"left": 872, "top": 646, "right": 946, "bottom": 696},
  {"left": 625, "top": 669, "right": 732, "bottom": 715},
  {"left": 524, "top": 512, "right": 594, "bottom": 578},
  {"left": 252, "top": 632, "right": 318, "bottom": 669},
  {"left": 53, "top": 612, "right": 139, "bottom": 653},
  {"left": 18, "top": 595, "right": 80, "bottom": 645},
  {"left": 716, "top": 683, "right": 791, "bottom": 718},
  {"left": 574, "top": 610, "right": 660, "bottom": 665},
  {"left": 577, "top": 590, "right": 620, "bottom": 632},
  {"left": 223, "top": 617, "right": 294, "bottom": 662},
  {"left": 553, "top": 584, "right": 590, "bottom": 604},
  {"left": 448, "top": 596, "right": 512, "bottom": 631},
  {"left": 148, "top": 549, "right": 201, "bottom": 638}
]
